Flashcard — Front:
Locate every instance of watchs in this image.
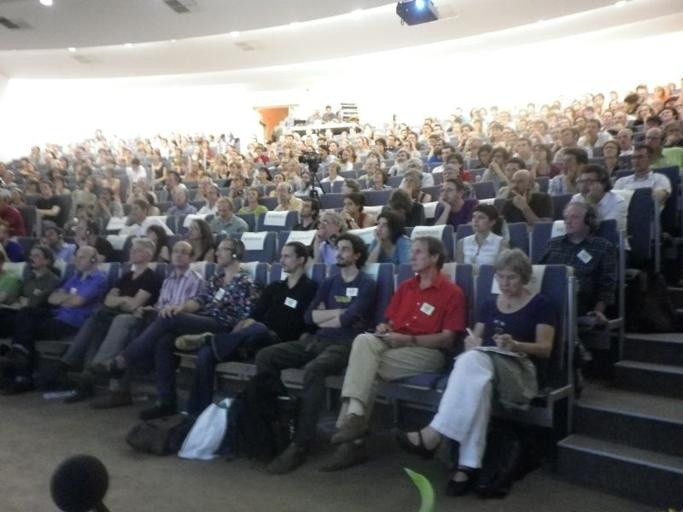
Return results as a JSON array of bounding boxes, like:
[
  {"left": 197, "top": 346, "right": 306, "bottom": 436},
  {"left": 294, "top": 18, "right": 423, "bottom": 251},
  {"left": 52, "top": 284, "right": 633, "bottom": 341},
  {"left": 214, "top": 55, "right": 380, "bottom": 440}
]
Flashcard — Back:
[{"left": 409, "top": 332, "right": 419, "bottom": 347}]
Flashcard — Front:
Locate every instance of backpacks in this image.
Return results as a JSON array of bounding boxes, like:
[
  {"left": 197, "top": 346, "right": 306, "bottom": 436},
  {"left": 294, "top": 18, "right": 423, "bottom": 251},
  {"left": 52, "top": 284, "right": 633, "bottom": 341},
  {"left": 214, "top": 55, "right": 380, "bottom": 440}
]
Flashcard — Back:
[
  {"left": 220, "top": 390, "right": 279, "bottom": 458},
  {"left": 126, "top": 413, "right": 197, "bottom": 455}
]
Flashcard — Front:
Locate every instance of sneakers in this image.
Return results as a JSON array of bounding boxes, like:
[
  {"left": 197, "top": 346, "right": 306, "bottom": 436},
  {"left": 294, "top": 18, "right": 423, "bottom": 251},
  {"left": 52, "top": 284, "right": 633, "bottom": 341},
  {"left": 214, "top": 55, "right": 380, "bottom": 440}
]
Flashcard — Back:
[
  {"left": 3, "top": 348, "right": 30, "bottom": 369},
  {"left": 328, "top": 412, "right": 372, "bottom": 445},
  {"left": 3, "top": 378, "right": 30, "bottom": 393},
  {"left": 316, "top": 441, "right": 363, "bottom": 473}
]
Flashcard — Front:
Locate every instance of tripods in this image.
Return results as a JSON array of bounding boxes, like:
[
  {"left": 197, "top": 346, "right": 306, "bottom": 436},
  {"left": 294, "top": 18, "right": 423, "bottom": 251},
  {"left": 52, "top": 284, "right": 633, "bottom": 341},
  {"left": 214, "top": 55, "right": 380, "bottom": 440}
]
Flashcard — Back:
[{"left": 302, "top": 173, "right": 326, "bottom": 198}]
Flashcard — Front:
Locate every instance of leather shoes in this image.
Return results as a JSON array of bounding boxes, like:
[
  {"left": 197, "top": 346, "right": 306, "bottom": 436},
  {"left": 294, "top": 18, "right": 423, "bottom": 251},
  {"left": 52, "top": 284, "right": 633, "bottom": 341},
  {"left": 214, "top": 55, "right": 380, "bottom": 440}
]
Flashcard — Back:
[
  {"left": 138, "top": 399, "right": 178, "bottom": 421},
  {"left": 444, "top": 467, "right": 474, "bottom": 496},
  {"left": 397, "top": 430, "right": 439, "bottom": 461},
  {"left": 66, "top": 365, "right": 112, "bottom": 389},
  {"left": 86, "top": 358, "right": 127, "bottom": 380},
  {"left": 276, "top": 437, "right": 312, "bottom": 474},
  {"left": 88, "top": 390, "right": 133, "bottom": 410},
  {"left": 59, "top": 387, "right": 97, "bottom": 404},
  {"left": 575, "top": 343, "right": 593, "bottom": 368}
]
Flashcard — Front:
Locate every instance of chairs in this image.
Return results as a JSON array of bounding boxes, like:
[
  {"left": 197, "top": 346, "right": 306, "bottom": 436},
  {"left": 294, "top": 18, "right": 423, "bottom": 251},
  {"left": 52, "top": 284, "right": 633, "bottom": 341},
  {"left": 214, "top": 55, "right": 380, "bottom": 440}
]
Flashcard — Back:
[
  {"left": 435, "top": 263, "right": 575, "bottom": 435},
  {"left": 14, "top": 237, "right": 40, "bottom": 256},
  {"left": 167, "top": 261, "right": 267, "bottom": 368},
  {"left": 163, "top": 234, "right": 182, "bottom": 251},
  {"left": 103, "top": 216, "right": 132, "bottom": 231},
  {"left": 457, "top": 220, "right": 532, "bottom": 264},
  {"left": 530, "top": 218, "right": 625, "bottom": 360},
  {"left": 335, "top": 262, "right": 474, "bottom": 423},
  {"left": 317, "top": 208, "right": 343, "bottom": 225},
  {"left": 277, "top": 228, "right": 318, "bottom": 259},
  {"left": 0, "top": 260, "right": 31, "bottom": 282},
  {"left": 148, "top": 217, "right": 177, "bottom": 234},
  {"left": 215, "top": 230, "right": 276, "bottom": 263},
  {"left": 217, "top": 263, "right": 326, "bottom": 381},
  {"left": 345, "top": 225, "right": 382, "bottom": 263},
  {"left": 479, "top": 200, "right": 499, "bottom": 210},
  {"left": 236, "top": 213, "right": 257, "bottom": 229},
  {"left": 256, "top": 211, "right": 297, "bottom": 230},
  {"left": 0, "top": 88, "right": 683, "bottom": 208},
  {"left": 553, "top": 194, "right": 572, "bottom": 221},
  {"left": 119, "top": 263, "right": 165, "bottom": 282},
  {"left": 176, "top": 213, "right": 213, "bottom": 233},
  {"left": 402, "top": 225, "right": 455, "bottom": 261},
  {"left": 61, "top": 234, "right": 78, "bottom": 250},
  {"left": 655, "top": 165, "right": 681, "bottom": 233},
  {"left": 281, "top": 262, "right": 393, "bottom": 412},
  {"left": 49, "top": 261, "right": 74, "bottom": 282},
  {"left": 18, "top": 204, "right": 37, "bottom": 237},
  {"left": 96, "top": 233, "right": 136, "bottom": 261},
  {"left": 570, "top": 188, "right": 655, "bottom": 295},
  {"left": 418, "top": 202, "right": 439, "bottom": 223},
  {"left": 34, "top": 261, "right": 119, "bottom": 359},
  {"left": 363, "top": 206, "right": 382, "bottom": 224},
  {"left": 161, "top": 262, "right": 215, "bottom": 279}
]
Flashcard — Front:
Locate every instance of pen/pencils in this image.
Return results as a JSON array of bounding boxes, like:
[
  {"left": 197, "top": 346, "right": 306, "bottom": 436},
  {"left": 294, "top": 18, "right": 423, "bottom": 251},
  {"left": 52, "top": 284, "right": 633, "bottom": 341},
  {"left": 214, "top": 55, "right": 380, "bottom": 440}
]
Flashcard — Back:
[
  {"left": 465, "top": 328, "right": 480, "bottom": 347},
  {"left": 384, "top": 320, "right": 395, "bottom": 334}
]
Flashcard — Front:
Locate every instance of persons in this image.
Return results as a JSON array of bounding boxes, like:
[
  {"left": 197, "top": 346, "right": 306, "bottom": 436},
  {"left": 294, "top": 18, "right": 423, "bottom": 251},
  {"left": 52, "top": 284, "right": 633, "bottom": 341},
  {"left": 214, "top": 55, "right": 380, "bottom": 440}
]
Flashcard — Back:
[
  {"left": 597, "top": 140, "right": 622, "bottom": 173},
  {"left": 513, "top": 136, "right": 536, "bottom": 163},
  {"left": 405, "top": 157, "right": 436, "bottom": 187},
  {"left": 0, "top": 144, "right": 43, "bottom": 187},
  {"left": 0, "top": 244, "right": 61, "bottom": 354},
  {"left": 576, "top": 119, "right": 613, "bottom": 158},
  {"left": 38, "top": 219, "right": 68, "bottom": 249},
  {"left": 216, "top": 132, "right": 275, "bottom": 192},
  {"left": 607, "top": 144, "right": 672, "bottom": 237},
  {"left": 104, "top": 195, "right": 173, "bottom": 252},
  {"left": 237, "top": 187, "right": 269, "bottom": 214},
  {"left": 396, "top": 168, "right": 432, "bottom": 204},
  {"left": 266, "top": 173, "right": 286, "bottom": 197},
  {"left": 490, "top": 168, "right": 555, "bottom": 223},
  {"left": 86, "top": 234, "right": 261, "bottom": 421},
  {"left": 198, "top": 185, "right": 221, "bottom": 214},
  {"left": 41, "top": 141, "right": 96, "bottom": 218},
  {"left": 535, "top": 146, "right": 561, "bottom": 176},
  {"left": 145, "top": 224, "right": 175, "bottom": 262},
  {"left": 448, "top": 106, "right": 517, "bottom": 162},
  {"left": 0, "top": 186, "right": 28, "bottom": 235},
  {"left": 339, "top": 192, "right": 376, "bottom": 231},
  {"left": 90, "top": 186, "right": 126, "bottom": 230},
  {"left": 453, "top": 201, "right": 507, "bottom": 278},
  {"left": 662, "top": 121, "right": 683, "bottom": 148},
  {"left": 346, "top": 114, "right": 422, "bottom": 170},
  {"left": 292, "top": 198, "right": 322, "bottom": 230},
  {"left": 546, "top": 146, "right": 588, "bottom": 196},
  {"left": 314, "top": 208, "right": 370, "bottom": 268},
  {"left": 304, "top": 105, "right": 347, "bottom": 126},
  {"left": 476, "top": 146, "right": 509, "bottom": 183},
  {"left": 643, "top": 125, "right": 682, "bottom": 168},
  {"left": 174, "top": 241, "right": 319, "bottom": 428},
  {"left": 0, "top": 219, "right": 26, "bottom": 259},
  {"left": 252, "top": 232, "right": 380, "bottom": 473},
  {"left": 67, "top": 239, "right": 205, "bottom": 410},
  {"left": 95, "top": 128, "right": 168, "bottom": 196},
  {"left": 392, "top": 246, "right": 561, "bottom": 499},
  {"left": 294, "top": 172, "right": 324, "bottom": 199},
  {"left": 616, "top": 128, "right": 635, "bottom": 157},
  {"left": 338, "top": 176, "right": 360, "bottom": 194},
  {"left": 360, "top": 210, "right": 411, "bottom": 264},
  {"left": 167, "top": 133, "right": 222, "bottom": 182},
  {"left": 494, "top": 157, "right": 525, "bottom": 199},
  {"left": 180, "top": 218, "right": 216, "bottom": 263},
  {"left": 443, "top": 153, "right": 475, "bottom": 181},
  {"left": 386, "top": 187, "right": 426, "bottom": 225},
  {"left": 319, "top": 162, "right": 347, "bottom": 182},
  {"left": 364, "top": 169, "right": 393, "bottom": 193},
  {"left": 563, "top": 163, "right": 633, "bottom": 253},
  {"left": 1, "top": 245, "right": 110, "bottom": 392},
  {"left": 207, "top": 196, "right": 250, "bottom": 237},
  {"left": 325, "top": 235, "right": 467, "bottom": 472},
  {"left": 273, "top": 128, "right": 354, "bottom": 172},
  {"left": 158, "top": 171, "right": 181, "bottom": 203},
  {"left": 517, "top": 101, "right": 574, "bottom": 147},
  {"left": 420, "top": 118, "right": 447, "bottom": 160},
  {"left": 270, "top": 181, "right": 307, "bottom": 217},
  {"left": 431, "top": 179, "right": 481, "bottom": 225},
  {"left": 476, "top": 143, "right": 495, "bottom": 169},
  {"left": 432, "top": 145, "right": 454, "bottom": 173},
  {"left": 559, "top": 82, "right": 682, "bottom": 122},
  {"left": 168, "top": 183, "right": 199, "bottom": 225},
  {"left": 72, "top": 217, "right": 115, "bottom": 262},
  {"left": 39, "top": 237, "right": 163, "bottom": 396},
  {"left": 544, "top": 196, "right": 618, "bottom": 401},
  {"left": 0, "top": 249, "right": 21, "bottom": 309},
  {"left": 442, "top": 165, "right": 478, "bottom": 199}
]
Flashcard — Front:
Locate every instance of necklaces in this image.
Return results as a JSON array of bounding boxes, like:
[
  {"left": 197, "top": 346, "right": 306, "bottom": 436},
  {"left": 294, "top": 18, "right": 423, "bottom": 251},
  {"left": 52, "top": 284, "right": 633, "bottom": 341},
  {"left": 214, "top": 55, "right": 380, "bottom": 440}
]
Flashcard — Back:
[{"left": 499, "top": 295, "right": 529, "bottom": 311}]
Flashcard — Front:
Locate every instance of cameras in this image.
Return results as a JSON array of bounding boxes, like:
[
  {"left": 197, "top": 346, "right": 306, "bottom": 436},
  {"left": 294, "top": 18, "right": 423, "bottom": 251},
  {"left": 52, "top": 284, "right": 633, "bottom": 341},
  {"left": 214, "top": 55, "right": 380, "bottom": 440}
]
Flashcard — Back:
[{"left": 299, "top": 153, "right": 321, "bottom": 173}]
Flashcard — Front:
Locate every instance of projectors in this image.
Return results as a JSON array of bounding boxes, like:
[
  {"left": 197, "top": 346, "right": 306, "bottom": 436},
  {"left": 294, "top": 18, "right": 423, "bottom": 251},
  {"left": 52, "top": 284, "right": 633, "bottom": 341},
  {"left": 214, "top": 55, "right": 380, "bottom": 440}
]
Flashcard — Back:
[{"left": 396, "top": 0, "right": 439, "bottom": 26}]
